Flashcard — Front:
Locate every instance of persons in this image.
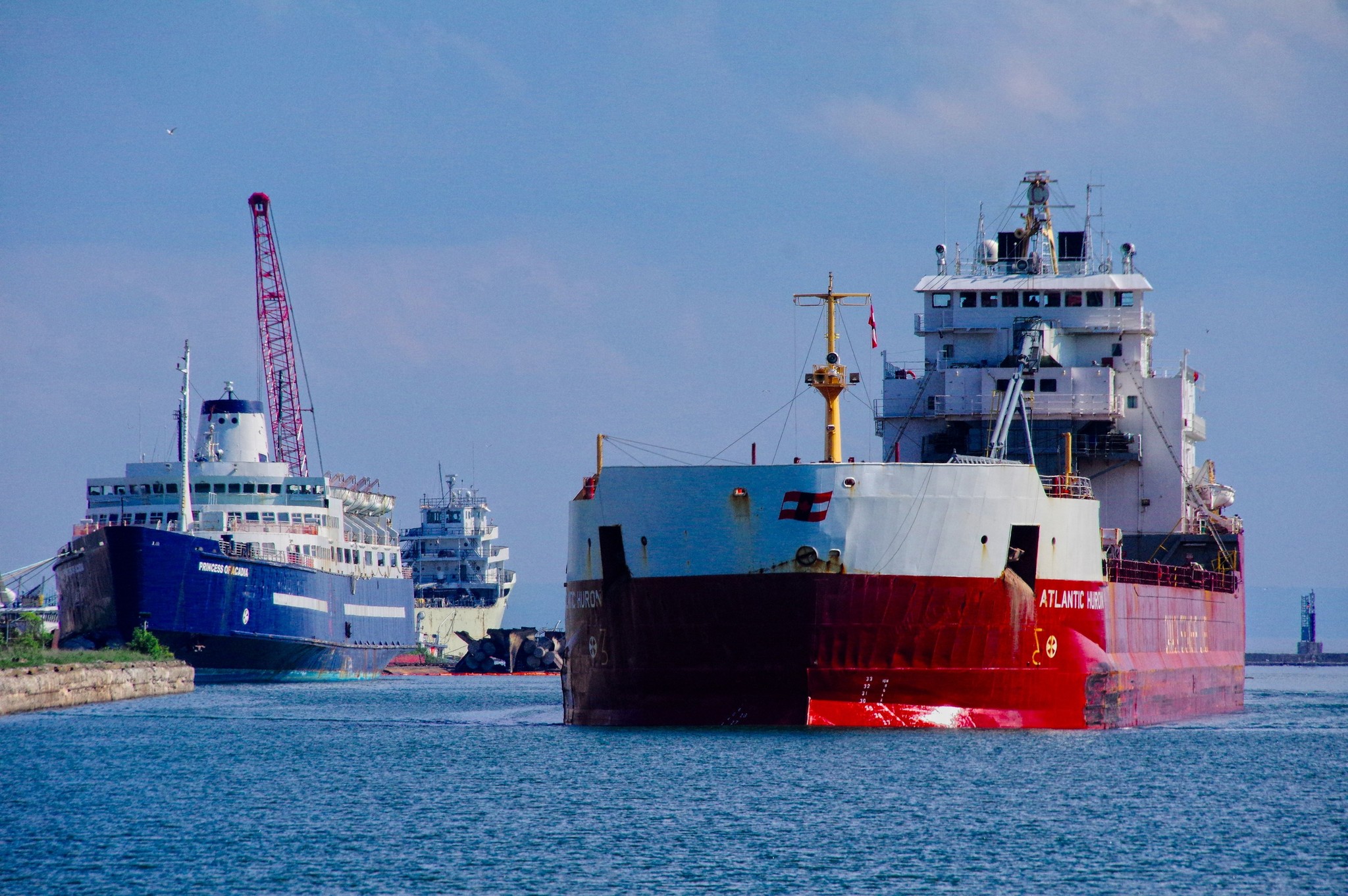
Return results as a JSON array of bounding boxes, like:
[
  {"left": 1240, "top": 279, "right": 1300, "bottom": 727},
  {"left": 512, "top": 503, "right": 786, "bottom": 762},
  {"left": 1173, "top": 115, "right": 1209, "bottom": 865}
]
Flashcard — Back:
[
  {"left": 1029, "top": 296, "right": 1038, "bottom": 303},
  {"left": 1091, "top": 360, "right": 1100, "bottom": 367}
]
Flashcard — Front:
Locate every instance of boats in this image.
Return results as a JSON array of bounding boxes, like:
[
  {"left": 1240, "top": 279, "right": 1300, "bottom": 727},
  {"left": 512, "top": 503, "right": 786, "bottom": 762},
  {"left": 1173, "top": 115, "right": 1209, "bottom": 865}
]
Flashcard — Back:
[
  {"left": 53, "top": 339, "right": 424, "bottom": 680},
  {"left": 401, "top": 459, "right": 523, "bottom": 661}
]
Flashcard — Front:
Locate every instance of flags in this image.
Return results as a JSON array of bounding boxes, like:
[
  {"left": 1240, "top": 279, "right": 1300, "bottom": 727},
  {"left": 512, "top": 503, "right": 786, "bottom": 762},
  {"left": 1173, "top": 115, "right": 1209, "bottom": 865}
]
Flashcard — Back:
[{"left": 868, "top": 304, "right": 877, "bottom": 348}]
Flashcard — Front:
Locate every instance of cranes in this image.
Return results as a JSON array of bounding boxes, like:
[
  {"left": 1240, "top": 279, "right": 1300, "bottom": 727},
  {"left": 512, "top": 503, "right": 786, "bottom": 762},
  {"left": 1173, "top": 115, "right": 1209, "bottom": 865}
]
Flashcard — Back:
[{"left": 248, "top": 191, "right": 326, "bottom": 476}]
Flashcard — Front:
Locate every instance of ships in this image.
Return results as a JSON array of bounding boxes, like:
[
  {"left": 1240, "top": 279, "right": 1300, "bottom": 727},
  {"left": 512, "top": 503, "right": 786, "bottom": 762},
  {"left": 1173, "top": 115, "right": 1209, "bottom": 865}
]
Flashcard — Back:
[{"left": 561, "top": 165, "right": 1255, "bottom": 732}]
